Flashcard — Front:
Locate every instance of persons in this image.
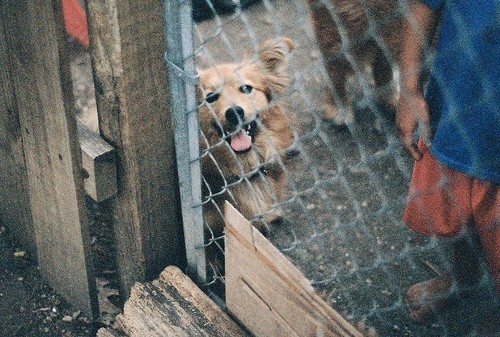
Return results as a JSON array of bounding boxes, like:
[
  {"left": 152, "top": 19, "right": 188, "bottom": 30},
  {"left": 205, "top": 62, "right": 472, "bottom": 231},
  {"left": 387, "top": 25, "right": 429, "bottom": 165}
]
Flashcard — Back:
[{"left": 392, "top": 0, "right": 500, "bottom": 321}]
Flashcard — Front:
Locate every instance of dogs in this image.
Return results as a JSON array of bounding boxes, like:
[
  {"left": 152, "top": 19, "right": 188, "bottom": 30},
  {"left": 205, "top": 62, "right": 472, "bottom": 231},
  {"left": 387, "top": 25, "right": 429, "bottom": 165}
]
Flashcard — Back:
[
  {"left": 309, "top": 0, "right": 410, "bottom": 128},
  {"left": 195, "top": 36, "right": 299, "bottom": 238}
]
